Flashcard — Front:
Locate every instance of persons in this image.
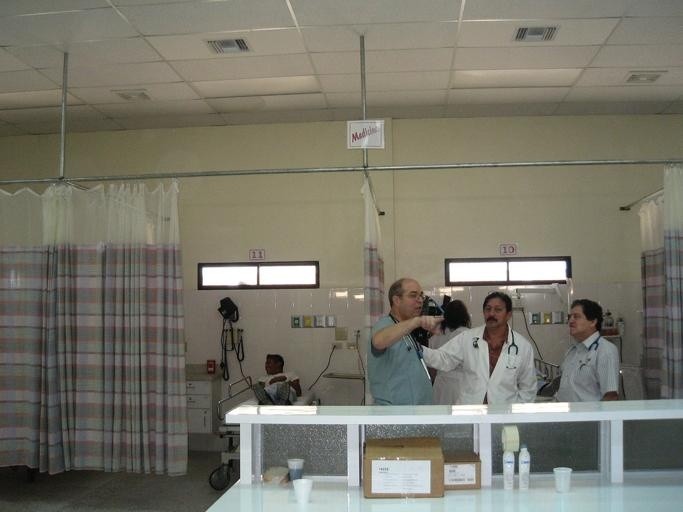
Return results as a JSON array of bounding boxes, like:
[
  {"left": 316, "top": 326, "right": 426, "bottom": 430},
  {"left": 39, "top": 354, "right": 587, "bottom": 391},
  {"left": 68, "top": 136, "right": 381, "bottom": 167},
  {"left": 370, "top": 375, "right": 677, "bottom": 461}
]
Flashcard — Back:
[
  {"left": 367, "top": 278, "right": 444, "bottom": 405},
  {"left": 549, "top": 300, "right": 621, "bottom": 471},
  {"left": 418, "top": 292, "right": 538, "bottom": 405},
  {"left": 253, "top": 354, "right": 301, "bottom": 404},
  {"left": 428, "top": 300, "right": 472, "bottom": 404}
]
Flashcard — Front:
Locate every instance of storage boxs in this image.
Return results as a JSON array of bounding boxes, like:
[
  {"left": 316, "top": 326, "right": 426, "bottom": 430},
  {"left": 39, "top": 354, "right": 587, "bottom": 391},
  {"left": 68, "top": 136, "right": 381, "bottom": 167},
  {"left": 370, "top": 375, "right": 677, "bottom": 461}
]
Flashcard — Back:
[
  {"left": 364, "top": 437, "right": 443, "bottom": 499},
  {"left": 443, "top": 452, "right": 481, "bottom": 490}
]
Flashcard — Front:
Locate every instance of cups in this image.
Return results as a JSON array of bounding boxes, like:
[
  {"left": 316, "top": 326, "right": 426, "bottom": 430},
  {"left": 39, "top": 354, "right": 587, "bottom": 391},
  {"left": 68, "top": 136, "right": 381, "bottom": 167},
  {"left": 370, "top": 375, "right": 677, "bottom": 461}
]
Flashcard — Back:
[
  {"left": 553, "top": 467, "right": 572, "bottom": 494},
  {"left": 286, "top": 459, "right": 313, "bottom": 506}
]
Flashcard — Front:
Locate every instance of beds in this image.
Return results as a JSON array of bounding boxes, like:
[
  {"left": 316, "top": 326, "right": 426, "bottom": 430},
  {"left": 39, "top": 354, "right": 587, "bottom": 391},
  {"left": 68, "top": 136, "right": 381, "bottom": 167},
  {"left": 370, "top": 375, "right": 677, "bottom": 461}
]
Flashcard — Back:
[
  {"left": 534, "top": 359, "right": 561, "bottom": 402},
  {"left": 211, "top": 376, "right": 319, "bottom": 490}
]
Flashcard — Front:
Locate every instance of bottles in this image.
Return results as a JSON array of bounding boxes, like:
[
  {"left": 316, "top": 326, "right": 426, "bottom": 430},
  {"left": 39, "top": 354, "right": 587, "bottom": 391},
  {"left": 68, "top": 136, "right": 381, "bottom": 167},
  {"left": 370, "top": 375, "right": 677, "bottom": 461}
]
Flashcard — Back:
[
  {"left": 605, "top": 308, "right": 625, "bottom": 336},
  {"left": 502, "top": 445, "right": 532, "bottom": 492}
]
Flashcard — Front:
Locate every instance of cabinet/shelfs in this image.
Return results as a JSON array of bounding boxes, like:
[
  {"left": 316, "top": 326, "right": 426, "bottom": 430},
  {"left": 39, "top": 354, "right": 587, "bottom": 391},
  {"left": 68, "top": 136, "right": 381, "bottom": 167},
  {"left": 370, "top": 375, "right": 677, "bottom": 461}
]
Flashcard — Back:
[{"left": 185, "top": 363, "right": 223, "bottom": 451}]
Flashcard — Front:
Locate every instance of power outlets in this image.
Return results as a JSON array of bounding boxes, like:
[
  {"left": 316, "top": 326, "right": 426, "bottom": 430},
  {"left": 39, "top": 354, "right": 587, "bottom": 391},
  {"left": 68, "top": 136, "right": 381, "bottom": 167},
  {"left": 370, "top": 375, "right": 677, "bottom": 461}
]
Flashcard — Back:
[
  {"left": 331, "top": 342, "right": 343, "bottom": 350},
  {"left": 353, "top": 328, "right": 361, "bottom": 336}
]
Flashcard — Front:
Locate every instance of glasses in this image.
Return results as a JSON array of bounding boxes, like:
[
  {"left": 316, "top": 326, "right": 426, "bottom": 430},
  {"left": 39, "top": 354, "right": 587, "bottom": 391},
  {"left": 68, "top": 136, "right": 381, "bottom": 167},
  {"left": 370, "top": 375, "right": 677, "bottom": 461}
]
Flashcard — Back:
[{"left": 397, "top": 293, "right": 426, "bottom": 301}]
